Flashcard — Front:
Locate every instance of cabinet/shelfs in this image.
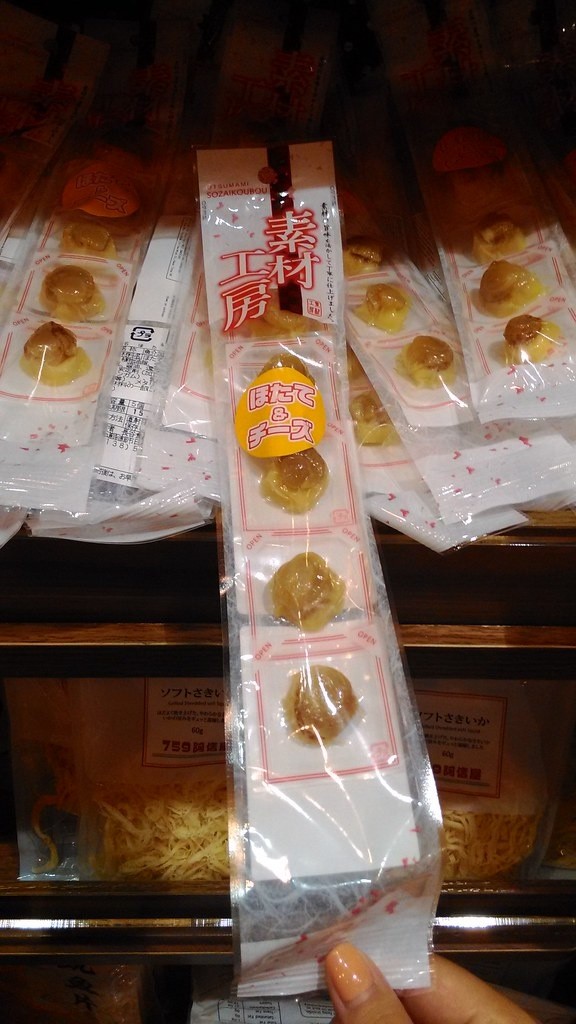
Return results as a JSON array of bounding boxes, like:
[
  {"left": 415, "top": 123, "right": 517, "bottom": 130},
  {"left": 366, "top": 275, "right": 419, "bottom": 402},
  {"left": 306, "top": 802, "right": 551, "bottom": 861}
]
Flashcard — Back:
[{"left": 0, "top": 511, "right": 576, "bottom": 965}]
